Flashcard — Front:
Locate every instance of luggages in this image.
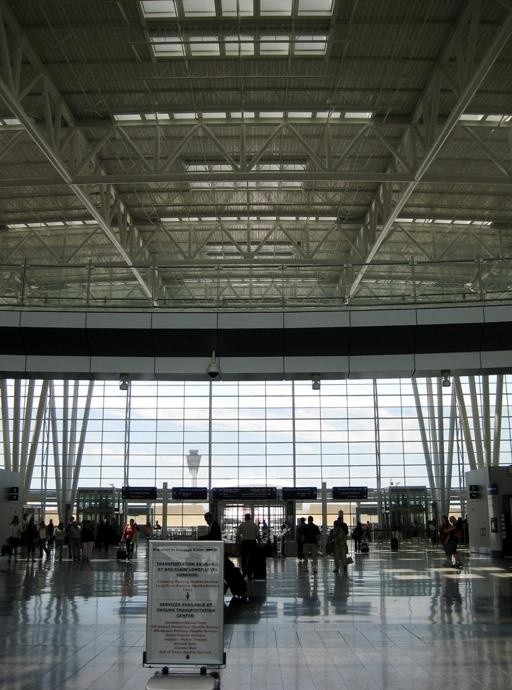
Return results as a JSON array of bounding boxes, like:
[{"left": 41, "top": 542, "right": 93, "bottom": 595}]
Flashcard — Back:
[
  {"left": 116, "top": 541, "right": 126, "bottom": 559},
  {"left": 390, "top": 538, "right": 399, "bottom": 552},
  {"left": 229, "top": 567, "right": 247, "bottom": 594},
  {"left": 360, "top": 539, "right": 369, "bottom": 553}
]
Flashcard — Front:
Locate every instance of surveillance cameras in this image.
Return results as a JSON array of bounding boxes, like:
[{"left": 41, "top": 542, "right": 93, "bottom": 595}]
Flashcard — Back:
[{"left": 207, "top": 363, "right": 219, "bottom": 378}]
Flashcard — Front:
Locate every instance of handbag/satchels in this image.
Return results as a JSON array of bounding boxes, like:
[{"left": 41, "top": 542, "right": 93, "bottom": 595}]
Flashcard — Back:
[
  {"left": 343, "top": 557, "right": 353, "bottom": 566},
  {"left": 325, "top": 541, "right": 335, "bottom": 555}
]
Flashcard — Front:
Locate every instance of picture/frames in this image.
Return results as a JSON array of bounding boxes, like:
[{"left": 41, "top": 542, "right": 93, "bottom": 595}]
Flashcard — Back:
[{"left": 146, "top": 539, "right": 224, "bottom": 665}]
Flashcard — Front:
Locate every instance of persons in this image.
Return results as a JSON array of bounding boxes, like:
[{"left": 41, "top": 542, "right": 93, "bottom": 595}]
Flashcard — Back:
[
  {"left": 7, "top": 512, "right": 162, "bottom": 562},
  {"left": 293, "top": 516, "right": 349, "bottom": 572},
  {"left": 352, "top": 521, "right": 372, "bottom": 552},
  {"left": 198, "top": 513, "right": 271, "bottom": 594},
  {"left": 431, "top": 515, "right": 468, "bottom": 569}
]
